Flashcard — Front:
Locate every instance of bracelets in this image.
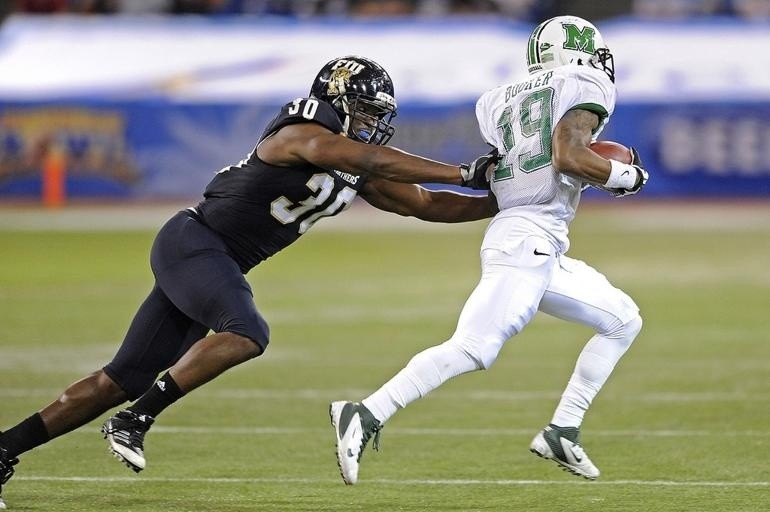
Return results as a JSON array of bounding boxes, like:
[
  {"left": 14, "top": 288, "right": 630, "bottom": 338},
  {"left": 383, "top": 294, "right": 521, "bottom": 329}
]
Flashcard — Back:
[{"left": 604, "top": 158, "right": 636, "bottom": 189}]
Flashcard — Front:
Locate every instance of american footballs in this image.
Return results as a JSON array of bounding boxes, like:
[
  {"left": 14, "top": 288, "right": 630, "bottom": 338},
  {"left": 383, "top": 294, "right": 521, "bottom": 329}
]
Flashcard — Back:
[{"left": 588, "top": 141, "right": 633, "bottom": 164}]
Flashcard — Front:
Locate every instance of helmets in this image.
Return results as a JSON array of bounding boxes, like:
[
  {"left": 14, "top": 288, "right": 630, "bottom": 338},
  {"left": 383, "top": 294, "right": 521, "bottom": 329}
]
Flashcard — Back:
[
  {"left": 527, "top": 15, "right": 615, "bottom": 84},
  {"left": 309, "top": 57, "right": 396, "bottom": 145}
]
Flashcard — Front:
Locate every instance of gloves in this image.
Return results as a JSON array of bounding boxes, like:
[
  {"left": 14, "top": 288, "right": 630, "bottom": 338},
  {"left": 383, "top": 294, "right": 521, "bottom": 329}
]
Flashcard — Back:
[
  {"left": 458, "top": 149, "right": 498, "bottom": 190},
  {"left": 603, "top": 146, "right": 649, "bottom": 198}
]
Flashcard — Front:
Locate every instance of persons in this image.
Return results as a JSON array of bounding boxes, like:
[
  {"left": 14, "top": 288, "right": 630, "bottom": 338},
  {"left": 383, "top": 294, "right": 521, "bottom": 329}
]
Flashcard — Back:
[
  {"left": 327, "top": 15, "right": 650, "bottom": 485},
  {"left": 1, "top": 55, "right": 505, "bottom": 511}
]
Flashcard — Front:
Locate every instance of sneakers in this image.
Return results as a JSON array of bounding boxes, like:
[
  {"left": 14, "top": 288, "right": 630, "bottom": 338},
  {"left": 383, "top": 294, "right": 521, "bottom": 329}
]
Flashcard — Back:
[
  {"left": 0, "top": 431, "right": 19, "bottom": 509},
  {"left": 529, "top": 423, "right": 601, "bottom": 479},
  {"left": 100, "top": 407, "right": 155, "bottom": 474},
  {"left": 330, "top": 401, "right": 383, "bottom": 486}
]
